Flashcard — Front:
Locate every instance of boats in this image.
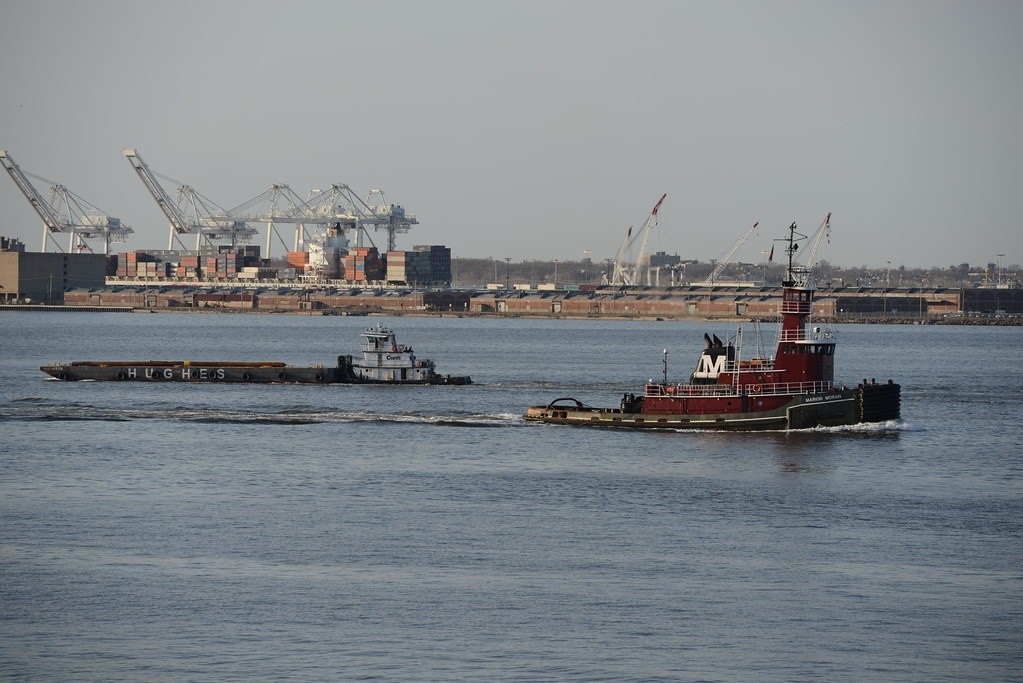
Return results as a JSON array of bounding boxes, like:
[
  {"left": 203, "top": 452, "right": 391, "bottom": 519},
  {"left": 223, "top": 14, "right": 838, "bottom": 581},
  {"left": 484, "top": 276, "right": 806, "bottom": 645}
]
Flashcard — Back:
[
  {"left": 516, "top": 219, "right": 903, "bottom": 436},
  {"left": 40, "top": 321, "right": 476, "bottom": 390}
]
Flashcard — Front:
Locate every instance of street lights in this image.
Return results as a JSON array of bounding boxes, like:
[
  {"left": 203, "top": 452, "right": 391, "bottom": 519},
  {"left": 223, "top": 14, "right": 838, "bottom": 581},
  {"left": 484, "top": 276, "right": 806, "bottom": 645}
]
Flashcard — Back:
[
  {"left": 604, "top": 258, "right": 614, "bottom": 286},
  {"left": 553, "top": 259, "right": 559, "bottom": 284},
  {"left": 584, "top": 248, "right": 591, "bottom": 282},
  {"left": 506, "top": 258, "right": 511, "bottom": 290},
  {"left": 997, "top": 254, "right": 1006, "bottom": 287}
]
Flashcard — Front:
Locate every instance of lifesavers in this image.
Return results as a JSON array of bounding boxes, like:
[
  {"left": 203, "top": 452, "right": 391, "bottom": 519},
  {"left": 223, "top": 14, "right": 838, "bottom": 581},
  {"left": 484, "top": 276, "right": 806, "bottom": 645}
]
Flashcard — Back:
[
  {"left": 208, "top": 370, "right": 218, "bottom": 381},
  {"left": 151, "top": 371, "right": 159, "bottom": 380},
  {"left": 278, "top": 372, "right": 286, "bottom": 381},
  {"left": 191, "top": 369, "right": 200, "bottom": 380},
  {"left": 752, "top": 385, "right": 762, "bottom": 392},
  {"left": 314, "top": 372, "right": 323, "bottom": 383},
  {"left": 241, "top": 371, "right": 251, "bottom": 382},
  {"left": 115, "top": 371, "right": 126, "bottom": 381},
  {"left": 58, "top": 372, "right": 67, "bottom": 380}
]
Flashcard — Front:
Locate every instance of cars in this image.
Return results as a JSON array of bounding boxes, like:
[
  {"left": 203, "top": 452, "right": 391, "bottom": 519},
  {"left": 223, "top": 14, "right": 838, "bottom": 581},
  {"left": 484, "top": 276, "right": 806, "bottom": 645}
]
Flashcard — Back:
[{"left": 945, "top": 312, "right": 1023, "bottom": 319}]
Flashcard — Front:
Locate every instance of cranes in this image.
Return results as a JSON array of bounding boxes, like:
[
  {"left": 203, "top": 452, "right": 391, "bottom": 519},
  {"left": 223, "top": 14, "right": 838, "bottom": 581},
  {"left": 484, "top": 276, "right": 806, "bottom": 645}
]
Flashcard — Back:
[
  {"left": 603, "top": 196, "right": 837, "bottom": 294},
  {"left": 124, "top": 146, "right": 421, "bottom": 278},
  {"left": 0, "top": 151, "right": 134, "bottom": 254}
]
